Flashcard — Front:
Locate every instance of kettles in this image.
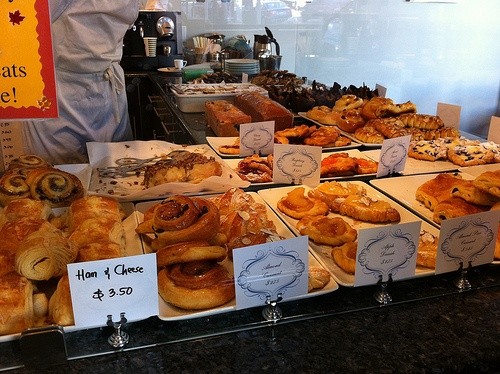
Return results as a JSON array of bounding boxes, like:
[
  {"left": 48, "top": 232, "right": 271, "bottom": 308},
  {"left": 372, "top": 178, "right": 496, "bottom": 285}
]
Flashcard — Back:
[{"left": 253, "top": 26, "right": 280, "bottom": 60}]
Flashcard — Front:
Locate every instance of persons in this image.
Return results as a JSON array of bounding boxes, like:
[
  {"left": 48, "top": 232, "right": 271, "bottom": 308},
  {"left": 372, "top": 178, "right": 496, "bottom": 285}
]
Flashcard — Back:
[{"left": 21, "top": 0, "right": 140, "bottom": 165}]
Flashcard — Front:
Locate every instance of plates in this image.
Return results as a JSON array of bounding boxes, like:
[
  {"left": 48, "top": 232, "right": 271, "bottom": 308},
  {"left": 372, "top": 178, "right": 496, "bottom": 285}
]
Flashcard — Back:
[
  {"left": 0, "top": 112, "right": 500, "bottom": 342},
  {"left": 157, "top": 67, "right": 182, "bottom": 72},
  {"left": 224, "top": 59, "right": 260, "bottom": 75}
]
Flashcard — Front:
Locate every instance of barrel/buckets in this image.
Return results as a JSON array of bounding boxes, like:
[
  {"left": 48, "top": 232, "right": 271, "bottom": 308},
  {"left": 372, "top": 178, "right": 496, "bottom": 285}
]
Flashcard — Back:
[{"left": 260, "top": 55, "right": 283, "bottom": 71}]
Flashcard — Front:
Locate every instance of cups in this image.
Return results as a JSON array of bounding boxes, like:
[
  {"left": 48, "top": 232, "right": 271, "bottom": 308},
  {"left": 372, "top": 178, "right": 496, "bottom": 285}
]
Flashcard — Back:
[
  {"left": 195, "top": 48, "right": 207, "bottom": 64},
  {"left": 143, "top": 37, "right": 157, "bottom": 57},
  {"left": 173, "top": 59, "right": 187, "bottom": 69}
]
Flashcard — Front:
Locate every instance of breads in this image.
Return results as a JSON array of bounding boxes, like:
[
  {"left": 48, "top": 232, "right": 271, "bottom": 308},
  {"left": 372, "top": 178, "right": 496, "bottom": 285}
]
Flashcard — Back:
[{"left": 0, "top": 92, "right": 500, "bottom": 335}]
